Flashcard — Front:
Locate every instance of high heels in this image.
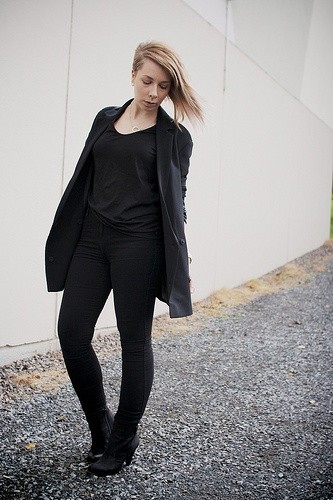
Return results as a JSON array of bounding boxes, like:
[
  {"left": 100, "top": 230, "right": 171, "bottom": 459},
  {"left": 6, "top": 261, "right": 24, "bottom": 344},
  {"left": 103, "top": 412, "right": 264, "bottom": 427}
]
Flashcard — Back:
[
  {"left": 88, "top": 409, "right": 114, "bottom": 462},
  {"left": 88, "top": 433, "right": 139, "bottom": 475}
]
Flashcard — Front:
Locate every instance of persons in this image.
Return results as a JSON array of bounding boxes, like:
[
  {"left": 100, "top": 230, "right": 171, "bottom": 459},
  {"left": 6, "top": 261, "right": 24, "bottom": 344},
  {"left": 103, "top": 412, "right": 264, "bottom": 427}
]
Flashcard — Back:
[{"left": 45, "top": 41, "right": 204, "bottom": 475}]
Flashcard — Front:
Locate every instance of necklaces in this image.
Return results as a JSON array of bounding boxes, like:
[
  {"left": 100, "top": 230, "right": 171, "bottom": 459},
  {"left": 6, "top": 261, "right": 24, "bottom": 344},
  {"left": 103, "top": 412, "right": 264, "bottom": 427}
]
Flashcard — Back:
[{"left": 129, "top": 100, "right": 158, "bottom": 131}]
